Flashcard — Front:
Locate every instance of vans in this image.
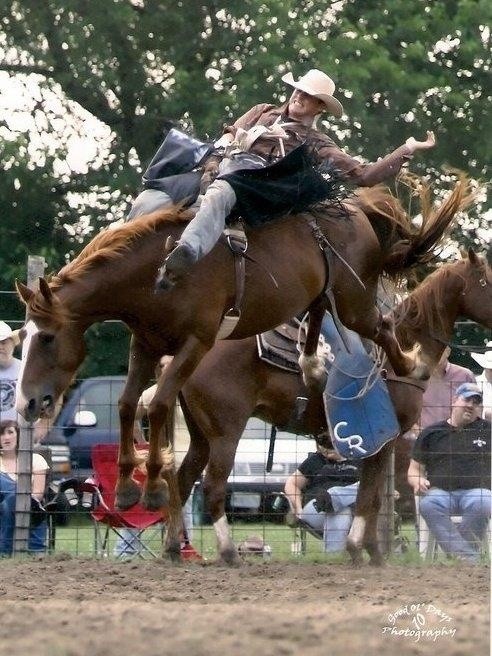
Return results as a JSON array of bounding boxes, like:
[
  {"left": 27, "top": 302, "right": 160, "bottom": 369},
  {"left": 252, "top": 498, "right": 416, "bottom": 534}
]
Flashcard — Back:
[{"left": 190, "top": 418, "right": 319, "bottom": 526}]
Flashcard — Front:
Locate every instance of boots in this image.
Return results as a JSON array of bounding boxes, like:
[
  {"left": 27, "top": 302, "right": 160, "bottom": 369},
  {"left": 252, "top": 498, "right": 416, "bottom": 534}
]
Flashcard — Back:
[{"left": 151, "top": 244, "right": 198, "bottom": 302}]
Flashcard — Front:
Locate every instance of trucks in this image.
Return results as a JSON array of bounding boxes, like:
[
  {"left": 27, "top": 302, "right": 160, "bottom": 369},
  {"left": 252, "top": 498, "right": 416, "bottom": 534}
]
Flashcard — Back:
[{"left": 38, "top": 375, "right": 139, "bottom": 470}]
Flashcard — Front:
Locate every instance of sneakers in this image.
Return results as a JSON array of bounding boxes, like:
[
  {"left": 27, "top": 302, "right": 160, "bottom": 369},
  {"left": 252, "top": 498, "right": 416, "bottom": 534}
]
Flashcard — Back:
[{"left": 314, "top": 487, "right": 333, "bottom": 515}]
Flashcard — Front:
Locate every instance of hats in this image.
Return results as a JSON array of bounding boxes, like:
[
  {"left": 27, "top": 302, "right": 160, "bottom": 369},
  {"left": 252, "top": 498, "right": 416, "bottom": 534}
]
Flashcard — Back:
[
  {"left": 455, "top": 383, "right": 483, "bottom": 399},
  {"left": 280, "top": 67, "right": 345, "bottom": 119},
  {"left": 0, "top": 322, "right": 20, "bottom": 345}
]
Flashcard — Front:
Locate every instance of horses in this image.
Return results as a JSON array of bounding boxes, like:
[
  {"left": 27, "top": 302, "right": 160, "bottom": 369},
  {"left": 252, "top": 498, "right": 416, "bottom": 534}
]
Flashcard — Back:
[
  {"left": 163, "top": 247, "right": 492, "bottom": 568},
  {"left": 14, "top": 174, "right": 481, "bottom": 512}
]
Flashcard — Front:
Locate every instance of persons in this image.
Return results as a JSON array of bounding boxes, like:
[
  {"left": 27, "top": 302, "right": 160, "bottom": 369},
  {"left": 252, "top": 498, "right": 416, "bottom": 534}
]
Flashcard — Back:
[
  {"left": 126, "top": 69, "right": 436, "bottom": 296},
  {"left": 112, "top": 355, "right": 199, "bottom": 561},
  {"left": 0, "top": 321, "right": 64, "bottom": 446},
  {"left": 285, "top": 443, "right": 400, "bottom": 552},
  {"left": 409, "top": 343, "right": 478, "bottom": 524},
  {"left": 407, "top": 384, "right": 492, "bottom": 562},
  {"left": 470, "top": 350, "right": 492, "bottom": 421}
]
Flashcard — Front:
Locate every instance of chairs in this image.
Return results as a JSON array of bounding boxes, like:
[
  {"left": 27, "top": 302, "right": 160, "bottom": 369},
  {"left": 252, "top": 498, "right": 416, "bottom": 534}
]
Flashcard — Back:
[
  {"left": 29, "top": 445, "right": 61, "bottom": 552},
  {"left": 414, "top": 470, "right": 491, "bottom": 563},
  {"left": 88, "top": 441, "right": 169, "bottom": 563},
  {"left": 293, "top": 452, "right": 397, "bottom": 561}
]
